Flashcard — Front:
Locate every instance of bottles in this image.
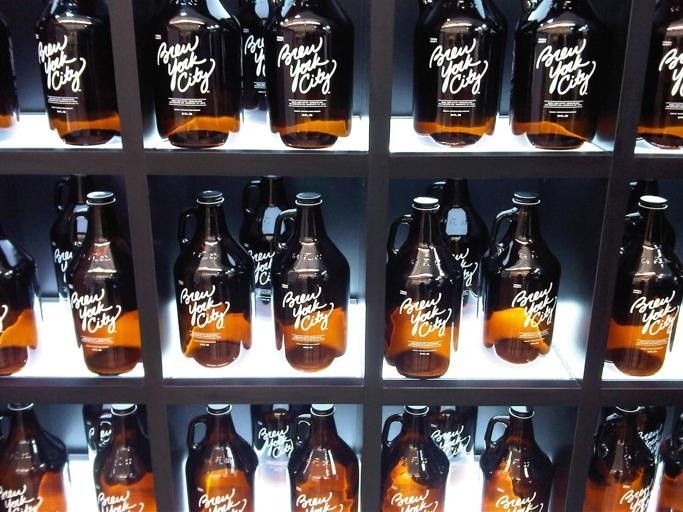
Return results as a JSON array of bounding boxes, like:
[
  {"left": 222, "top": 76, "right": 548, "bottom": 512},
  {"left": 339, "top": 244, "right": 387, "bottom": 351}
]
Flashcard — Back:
[
  {"left": 1, "top": 6, "right": 22, "bottom": 133},
  {"left": 81, "top": 402, "right": 158, "bottom": 510},
  {"left": 477, "top": 407, "right": 554, "bottom": 511},
  {"left": 383, "top": 174, "right": 488, "bottom": 380},
  {"left": 248, "top": 179, "right": 352, "bottom": 374},
  {"left": 636, "top": 10, "right": 683, "bottom": 154},
  {"left": 151, "top": 0, "right": 241, "bottom": 149},
  {"left": 384, "top": 404, "right": 482, "bottom": 510},
  {"left": 608, "top": 191, "right": 683, "bottom": 378},
  {"left": 2, "top": 235, "right": 38, "bottom": 377},
  {"left": 511, "top": 3, "right": 604, "bottom": 147},
  {"left": 482, "top": 191, "right": 569, "bottom": 366},
  {"left": 46, "top": 175, "right": 145, "bottom": 375},
  {"left": 171, "top": 190, "right": 257, "bottom": 369},
  {"left": 182, "top": 400, "right": 259, "bottom": 511},
  {"left": 578, "top": 390, "right": 683, "bottom": 512},
  {"left": 1, "top": 398, "right": 75, "bottom": 512},
  {"left": 407, "top": 2, "right": 506, "bottom": 146},
  {"left": 240, "top": 0, "right": 354, "bottom": 151},
  {"left": 256, "top": 403, "right": 359, "bottom": 512},
  {"left": 34, "top": 1, "right": 120, "bottom": 144}
]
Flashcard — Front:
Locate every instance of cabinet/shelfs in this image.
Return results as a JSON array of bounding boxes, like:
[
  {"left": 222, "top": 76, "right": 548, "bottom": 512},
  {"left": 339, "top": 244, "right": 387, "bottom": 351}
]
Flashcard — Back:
[{"left": 0, "top": 0, "right": 683, "bottom": 512}]
